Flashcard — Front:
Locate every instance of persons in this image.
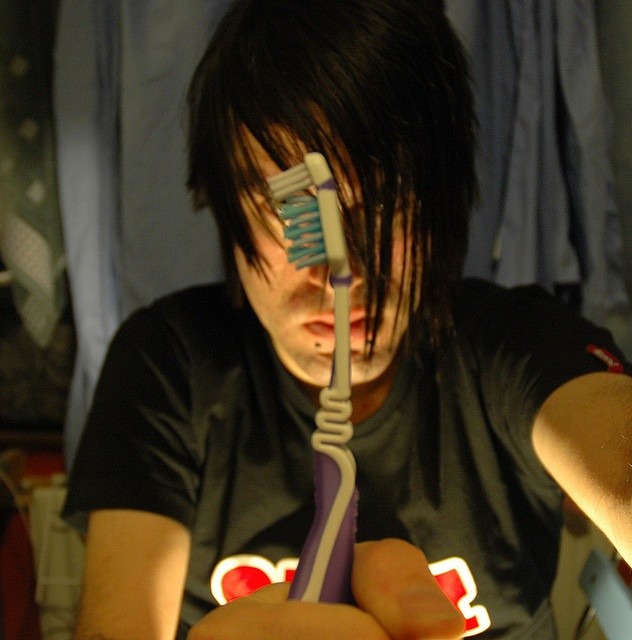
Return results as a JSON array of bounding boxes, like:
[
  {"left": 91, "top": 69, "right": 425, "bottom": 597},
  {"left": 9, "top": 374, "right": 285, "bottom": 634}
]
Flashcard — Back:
[{"left": 57, "top": 1, "right": 632, "bottom": 639}]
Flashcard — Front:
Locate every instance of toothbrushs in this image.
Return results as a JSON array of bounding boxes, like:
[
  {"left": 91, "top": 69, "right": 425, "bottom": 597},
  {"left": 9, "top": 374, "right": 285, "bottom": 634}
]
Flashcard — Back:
[{"left": 264, "top": 152, "right": 359, "bottom": 603}]
